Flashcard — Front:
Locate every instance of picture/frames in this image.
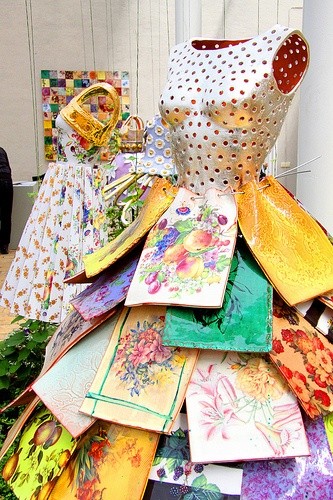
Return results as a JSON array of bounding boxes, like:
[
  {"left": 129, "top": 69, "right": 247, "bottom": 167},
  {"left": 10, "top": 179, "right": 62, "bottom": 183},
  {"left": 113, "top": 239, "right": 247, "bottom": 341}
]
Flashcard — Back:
[{"left": 40, "top": 70, "right": 131, "bottom": 162}]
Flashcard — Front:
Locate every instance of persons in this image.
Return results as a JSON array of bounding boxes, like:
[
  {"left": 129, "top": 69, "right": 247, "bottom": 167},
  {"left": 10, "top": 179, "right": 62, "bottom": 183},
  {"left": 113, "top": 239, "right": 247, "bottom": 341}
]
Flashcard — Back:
[{"left": 0, "top": 146, "right": 14, "bottom": 255}]
